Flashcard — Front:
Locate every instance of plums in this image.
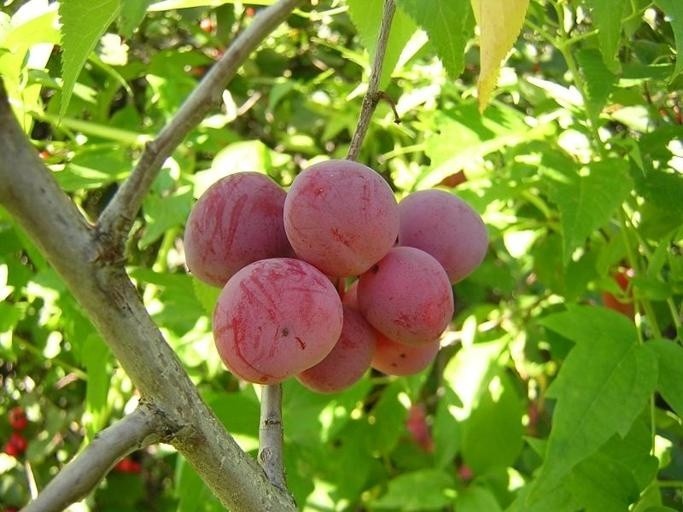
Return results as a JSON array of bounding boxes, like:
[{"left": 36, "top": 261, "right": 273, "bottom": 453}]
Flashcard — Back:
[{"left": 0, "top": 6, "right": 683, "bottom": 512}]
